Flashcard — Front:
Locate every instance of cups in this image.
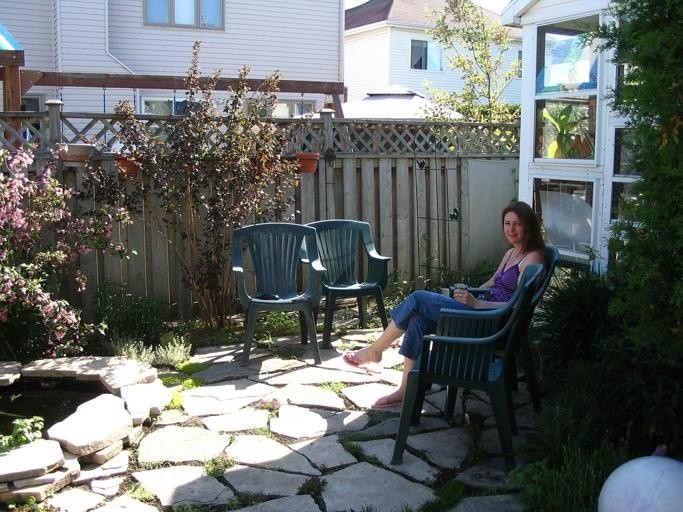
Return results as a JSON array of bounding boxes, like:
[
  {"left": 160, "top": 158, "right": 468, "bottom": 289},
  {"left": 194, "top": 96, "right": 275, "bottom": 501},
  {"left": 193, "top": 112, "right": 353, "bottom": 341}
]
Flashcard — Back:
[{"left": 454, "top": 283, "right": 469, "bottom": 290}]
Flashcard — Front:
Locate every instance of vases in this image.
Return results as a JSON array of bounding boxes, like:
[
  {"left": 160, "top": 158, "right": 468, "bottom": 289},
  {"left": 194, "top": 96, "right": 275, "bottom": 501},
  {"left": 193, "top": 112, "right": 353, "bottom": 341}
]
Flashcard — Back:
[
  {"left": 55, "top": 143, "right": 95, "bottom": 168},
  {"left": 115, "top": 154, "right": 139, "bottom": 181},
  {"left": 295, "top": 152, "right": 320, "bottom": 173}
]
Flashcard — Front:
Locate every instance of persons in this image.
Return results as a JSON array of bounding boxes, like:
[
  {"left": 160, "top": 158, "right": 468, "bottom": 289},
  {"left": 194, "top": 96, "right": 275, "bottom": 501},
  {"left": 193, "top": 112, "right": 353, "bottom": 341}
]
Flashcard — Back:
[{"left": 344, "top": 202, "right": 550, "bottom": 409}]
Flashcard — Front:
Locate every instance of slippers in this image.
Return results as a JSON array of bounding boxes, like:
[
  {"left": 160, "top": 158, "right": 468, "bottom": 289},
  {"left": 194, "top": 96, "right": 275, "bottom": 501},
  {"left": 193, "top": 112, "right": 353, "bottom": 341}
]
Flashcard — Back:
[
  {"left": 344, "top": 352, "right": 383, "bottom": 374},
  {"left": 372, "top": 395, "right": 402, "bottom": 408}
]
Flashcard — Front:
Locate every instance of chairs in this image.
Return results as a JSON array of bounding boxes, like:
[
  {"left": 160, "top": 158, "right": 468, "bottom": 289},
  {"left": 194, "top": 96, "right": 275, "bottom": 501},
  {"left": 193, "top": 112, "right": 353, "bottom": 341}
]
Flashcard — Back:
[
  {"left": 299, "top": 219, "right": 391, "bottom": 349},
  {"left": 232, "top": 222, "right": 327, "bottom": 367},
  {"left": 425, "top": 246, "right": 560, "bottom": 421},
  {"left": 390, "top": 262, "right": 544, "bottom": 464}
]
